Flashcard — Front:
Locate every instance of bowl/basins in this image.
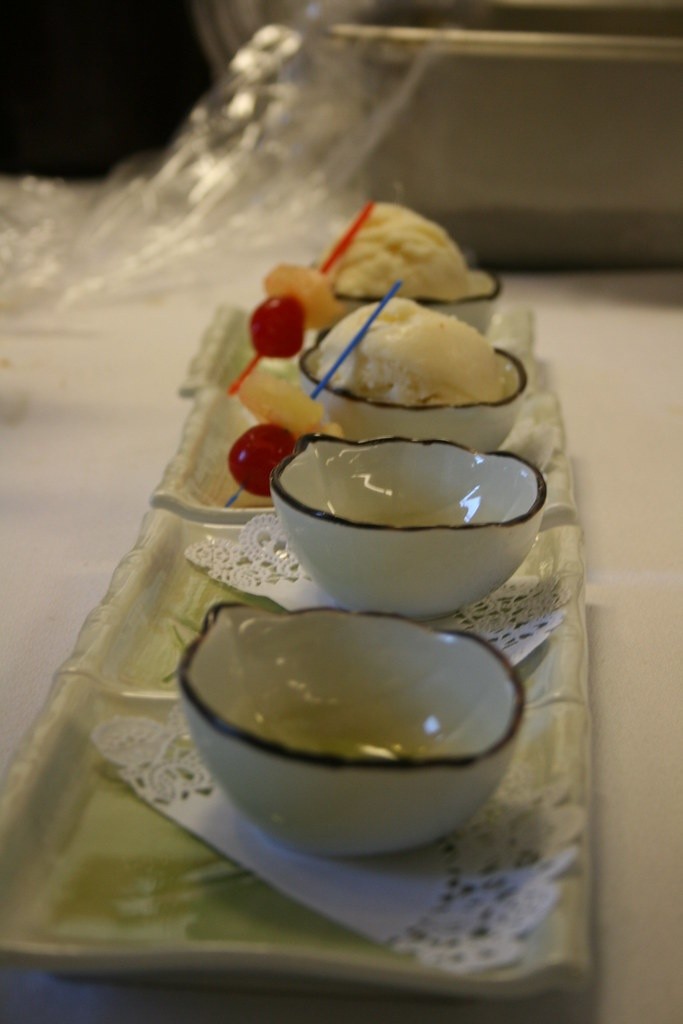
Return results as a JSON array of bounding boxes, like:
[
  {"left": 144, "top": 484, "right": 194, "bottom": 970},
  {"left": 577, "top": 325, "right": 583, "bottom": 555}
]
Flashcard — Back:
[
  {"left": 335, "top": 267, "right": 502, "bottom": 333},
  {"left": 181, "top": 603, "right": 524, "bottom": 856},
  {"left": 270, "top": 436, "right": 549, "bottom": 623},
  {"left": 301, "top": 327, "right": 529, "bottom": 448}
]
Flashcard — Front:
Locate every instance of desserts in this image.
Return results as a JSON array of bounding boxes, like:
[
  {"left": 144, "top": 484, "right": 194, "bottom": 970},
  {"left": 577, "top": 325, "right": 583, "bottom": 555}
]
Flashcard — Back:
[
  {"left": 311, "top": 295, "right": 512, "bottom": 408},
  {"left": 316, "top": 201, "right": 483, "bottom": 299}
]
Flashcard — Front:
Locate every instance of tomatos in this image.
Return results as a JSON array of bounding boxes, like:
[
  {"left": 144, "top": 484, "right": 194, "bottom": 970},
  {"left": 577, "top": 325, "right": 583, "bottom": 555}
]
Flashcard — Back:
[
  {"left": 250, "top": 293, "right": 307, "bottom": 358},
  {"left": 228, "top": 424, "right": 300, "bottom": 497}
]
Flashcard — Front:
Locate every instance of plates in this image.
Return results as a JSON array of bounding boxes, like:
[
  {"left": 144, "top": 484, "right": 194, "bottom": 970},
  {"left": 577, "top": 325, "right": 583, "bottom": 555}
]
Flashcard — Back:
[{"left": 0, "top": 305, "right": 594, "bottom": 999}]
